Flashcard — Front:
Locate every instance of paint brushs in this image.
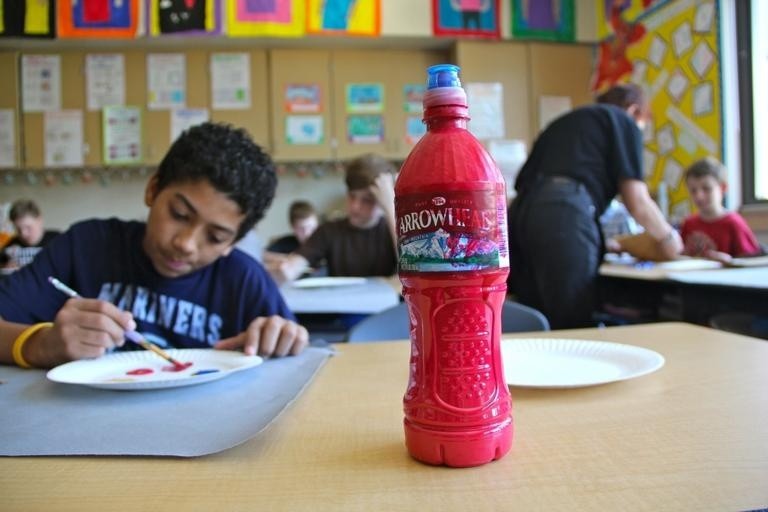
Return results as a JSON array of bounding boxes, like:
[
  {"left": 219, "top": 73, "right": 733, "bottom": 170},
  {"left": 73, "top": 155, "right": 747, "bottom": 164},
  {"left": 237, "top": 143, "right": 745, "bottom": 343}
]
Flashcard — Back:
[
  {"left": 264, "top": 255, "right": 320, "bottom": 273},
  {"left": 47, "top": 276, "right": 185, "bottom": 369}
]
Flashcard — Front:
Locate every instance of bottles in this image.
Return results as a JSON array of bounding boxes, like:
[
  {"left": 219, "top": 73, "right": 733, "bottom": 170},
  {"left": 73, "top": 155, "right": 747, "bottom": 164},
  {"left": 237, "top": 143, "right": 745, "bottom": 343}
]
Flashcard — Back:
[{"left": 391, "top": 63, "right": 517, "bottom": 468}]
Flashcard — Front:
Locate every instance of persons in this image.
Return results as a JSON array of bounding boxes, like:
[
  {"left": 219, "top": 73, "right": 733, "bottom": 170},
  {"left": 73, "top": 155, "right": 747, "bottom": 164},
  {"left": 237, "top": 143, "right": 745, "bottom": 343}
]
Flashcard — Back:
[
  {"left": 673, "top": 156, "right": 763, "bottom": 261},
  {"left": 506, "top": 81, "right": 685, "bottom": 337},
  {"left": 0, "top": 197, "right": 65, "bottom": 269},
  {"left": 0, "top": 120, "right": 309, "bottom": 370},
  {"left": 263, "top": 152, "right": 399, "bottom": 286},
  {"left": 261, "top": 199, "right": 328, "bottom": 278}
]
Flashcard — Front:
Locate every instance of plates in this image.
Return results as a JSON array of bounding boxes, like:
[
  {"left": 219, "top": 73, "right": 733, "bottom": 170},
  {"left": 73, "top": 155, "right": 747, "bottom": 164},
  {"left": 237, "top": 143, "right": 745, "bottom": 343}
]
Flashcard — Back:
[
  {"left": 47, "top": 347, "right": 264, "bottom": 391},
  {"left": 496, "top": 338, "right": 666, "bottom": 389}
]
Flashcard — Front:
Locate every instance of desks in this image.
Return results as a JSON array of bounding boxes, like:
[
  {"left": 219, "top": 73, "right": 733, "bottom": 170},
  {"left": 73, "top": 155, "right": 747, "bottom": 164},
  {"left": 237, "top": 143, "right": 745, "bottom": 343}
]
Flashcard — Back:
[
  {"left": 596, "top": 247, "right": 768, "bottom": 339},
  {"left": 279, "top": 271, "right": 401, "bottom": 315},
  {"left": 1, "top": 316, "right": 768, "bottom": 510}
]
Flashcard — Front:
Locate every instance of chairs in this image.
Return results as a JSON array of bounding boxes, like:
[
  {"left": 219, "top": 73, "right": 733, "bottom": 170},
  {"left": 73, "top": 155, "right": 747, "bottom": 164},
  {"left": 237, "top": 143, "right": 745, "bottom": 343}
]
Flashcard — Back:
[{"left": 347, "top": 297, "right": 553, "bottom": 342}]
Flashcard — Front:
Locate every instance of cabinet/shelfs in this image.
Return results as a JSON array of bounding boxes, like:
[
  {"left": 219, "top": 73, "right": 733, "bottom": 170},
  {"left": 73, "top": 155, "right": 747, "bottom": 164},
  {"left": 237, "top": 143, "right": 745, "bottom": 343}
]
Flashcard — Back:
[
  {"left": 269, "top": 43, "right": 452, "bottom": 166},
  {"left": 19, "top": 50, "right": 270, "bottom": 172},
  {"left": 1, "top": 49, "right": 24, "bottom": 173},
  {"left": 452, "top": 37, "right": 599, "bottom": 154}
]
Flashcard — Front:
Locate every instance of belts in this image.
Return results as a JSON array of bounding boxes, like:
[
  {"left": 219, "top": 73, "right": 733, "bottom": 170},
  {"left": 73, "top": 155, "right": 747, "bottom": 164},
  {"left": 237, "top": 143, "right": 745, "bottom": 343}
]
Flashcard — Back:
[{"left": 517, "top": 176, "right": 600, "bottom": 218}]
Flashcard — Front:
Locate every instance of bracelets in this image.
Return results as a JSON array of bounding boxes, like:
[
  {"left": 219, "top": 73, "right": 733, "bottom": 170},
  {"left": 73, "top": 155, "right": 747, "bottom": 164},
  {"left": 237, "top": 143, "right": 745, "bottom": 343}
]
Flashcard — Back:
[
  {"left": 9, "top": 321, "right": 55, "bottom": 370},
  {"left": 661, "top": 227, "right": 678, "bottom": 242}
]
